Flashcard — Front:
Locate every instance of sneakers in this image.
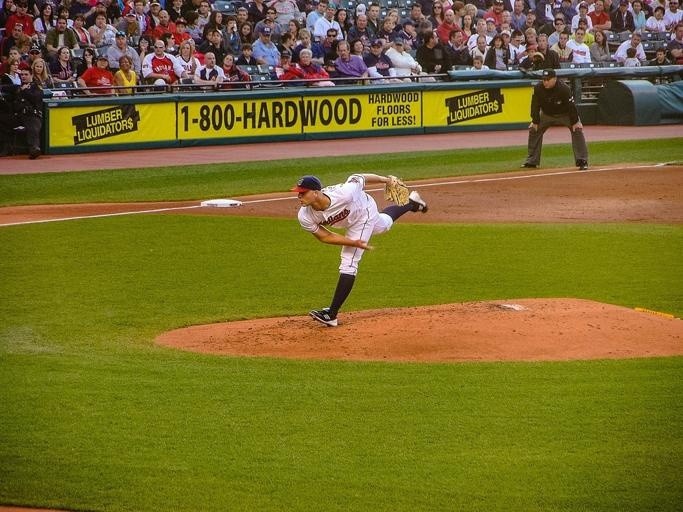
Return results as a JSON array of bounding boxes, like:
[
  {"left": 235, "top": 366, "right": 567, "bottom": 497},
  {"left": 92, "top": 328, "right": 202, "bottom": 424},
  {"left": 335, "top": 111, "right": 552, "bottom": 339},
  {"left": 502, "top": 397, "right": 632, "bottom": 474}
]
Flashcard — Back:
[
  {"left": 29, "top": 147, "right": 41, "bottom": 159},
  {"left": 576, "top": 159, "right": 588, "bottom": 169},
  {"left": 309, "top": 308, "right": 337, "bottom": 326},
  {"left": 520, "top": 163, "right": 536, "bottom": 168},
  {"left": 408, "top": 191, "right": 427, "bottom": 213}
]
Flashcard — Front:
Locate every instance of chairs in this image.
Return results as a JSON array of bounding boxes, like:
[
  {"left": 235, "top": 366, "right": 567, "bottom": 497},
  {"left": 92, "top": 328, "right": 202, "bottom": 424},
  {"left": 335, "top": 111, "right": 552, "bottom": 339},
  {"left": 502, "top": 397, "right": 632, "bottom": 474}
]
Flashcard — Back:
[{"left": 8, "top": 110, "right": 43, "bottom": 156}]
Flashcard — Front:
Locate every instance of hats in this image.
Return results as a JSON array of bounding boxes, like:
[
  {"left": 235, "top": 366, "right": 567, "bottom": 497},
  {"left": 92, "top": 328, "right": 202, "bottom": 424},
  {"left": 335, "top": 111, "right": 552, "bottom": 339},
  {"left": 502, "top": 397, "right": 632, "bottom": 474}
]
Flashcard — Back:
[
  {"left": 543, "top": 69, "right": 556, "bottom": 79},
  {"left": 291, "top": 176, "right": 321, "bottom": 192}
]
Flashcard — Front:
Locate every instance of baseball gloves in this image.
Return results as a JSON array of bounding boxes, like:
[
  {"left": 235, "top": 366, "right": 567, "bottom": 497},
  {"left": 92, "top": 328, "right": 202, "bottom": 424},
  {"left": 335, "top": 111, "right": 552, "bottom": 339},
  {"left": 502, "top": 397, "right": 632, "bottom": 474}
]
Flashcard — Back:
[{"left": 385, "top": 174, "right": 409, "bottom": 207}]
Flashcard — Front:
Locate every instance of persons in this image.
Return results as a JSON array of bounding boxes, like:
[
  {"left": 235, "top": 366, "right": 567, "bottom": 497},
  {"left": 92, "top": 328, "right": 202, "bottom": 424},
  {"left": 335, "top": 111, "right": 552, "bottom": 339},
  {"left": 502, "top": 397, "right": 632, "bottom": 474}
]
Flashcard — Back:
[
  {"left": 0, "top": 1, "right": 682, "bottom": 161},
  {"left": 289, "top": 174, "right": 428, "bottom": 325},
  {"left": 519, "top": 68, "right": 589, "bottom": 169}
]
